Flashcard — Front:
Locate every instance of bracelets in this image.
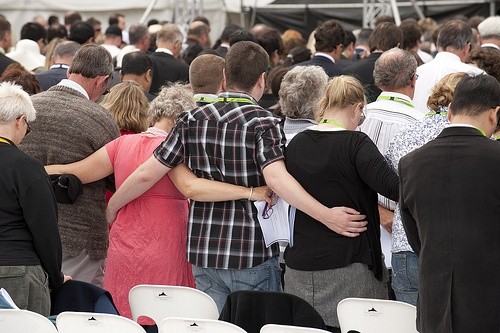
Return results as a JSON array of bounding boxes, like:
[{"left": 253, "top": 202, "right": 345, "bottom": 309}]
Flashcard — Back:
[{"left": 248, "top": 188, "right": 252, "bottom": 200}]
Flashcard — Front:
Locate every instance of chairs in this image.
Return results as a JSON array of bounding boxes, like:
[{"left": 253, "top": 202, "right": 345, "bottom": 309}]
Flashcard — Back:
[
  {"left": 337, "top": 297, "right": 419, "bottom": 333},
  {"left": 56, "top": 311, "right": 147, "bottom": 333},
  {"left": 128, "top": 283, "right": 221, "bottom": 333},
  {"left": 0, "top": 309, "right": 59, "bottom": 333},
  {"left": 218, "top": 290, "right": 335, "bottom": 333},
  {"left": 159, "top": 316, "right": 247, "bottom": 333},
  {"left": 57, "top": 280, "right": 122, "bottom": 315}
]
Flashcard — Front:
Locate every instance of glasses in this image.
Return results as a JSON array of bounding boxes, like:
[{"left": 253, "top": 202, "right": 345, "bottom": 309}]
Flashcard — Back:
[
  {"left": 351, "top": 103, "right": 366, "bottom": 127},
  {"left": 262, "top": 191, "right": 280, "bottom": 220},
  {"left": 410, "top": 73, "right": 419, "bottom": 80},
  {"left": 16, "top": 117, "right": 31, "bottom": 135}
]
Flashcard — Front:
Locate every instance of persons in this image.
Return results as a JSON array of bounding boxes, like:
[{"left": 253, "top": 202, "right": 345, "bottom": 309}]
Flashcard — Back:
[
  {"left": 378, "top": 73, "right": 468, "bottom": 307},
  {"left": 399, "top": 72, "right": 500, "bottom": 333},
  {"left": 0, "top": 11, "right": 500, "bottom": 327}
]
